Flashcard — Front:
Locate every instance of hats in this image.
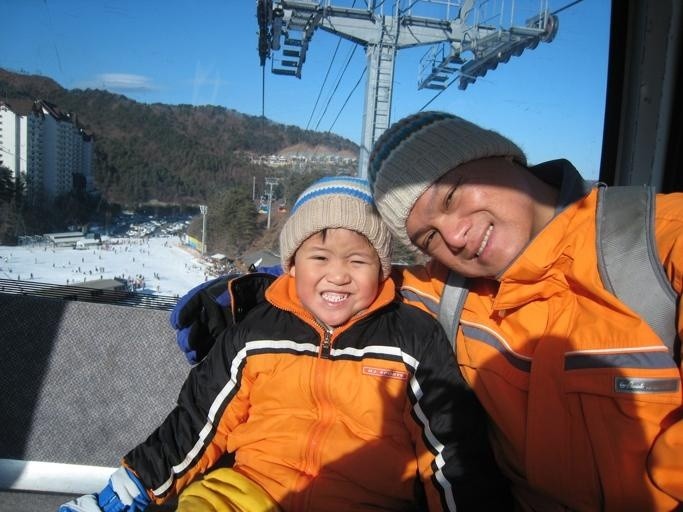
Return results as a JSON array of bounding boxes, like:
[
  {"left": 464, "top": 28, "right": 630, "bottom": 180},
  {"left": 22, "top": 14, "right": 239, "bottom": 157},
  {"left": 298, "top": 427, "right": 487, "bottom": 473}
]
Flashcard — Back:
[
  {"left": 279, "top": 177, "right": 393, "bottom": 284},
  {"left": 367, "top": 110, "right": 528, "bottom": 266}
]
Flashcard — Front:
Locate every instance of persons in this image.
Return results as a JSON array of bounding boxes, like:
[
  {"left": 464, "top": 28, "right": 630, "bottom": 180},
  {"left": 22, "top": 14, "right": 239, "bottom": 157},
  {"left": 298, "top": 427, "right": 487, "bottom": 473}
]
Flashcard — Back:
[
  {"left": 170, "top": 110, "right": 682, "bottom": 512},
  {"left": 59, "top": 177, "right": 516, "bottom": 511},
  {"left": 0, "top": 213, "right": 236, "bottom": 296}
]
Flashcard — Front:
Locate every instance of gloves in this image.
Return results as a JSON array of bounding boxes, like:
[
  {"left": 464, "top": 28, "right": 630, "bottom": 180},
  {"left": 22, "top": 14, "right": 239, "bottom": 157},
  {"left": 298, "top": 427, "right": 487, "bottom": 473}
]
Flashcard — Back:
[
  {"left": 171, "top": 266, "right": 282, "bottom": 364},
  {"left": 54, "top": 467, "right": 153, "bottom": 512}
]
